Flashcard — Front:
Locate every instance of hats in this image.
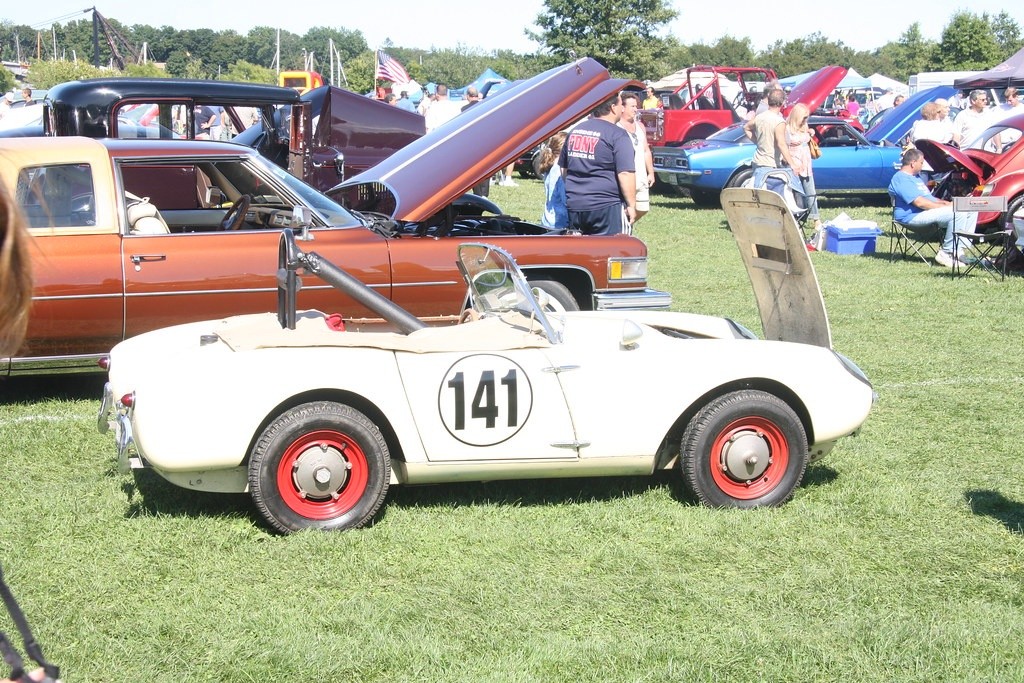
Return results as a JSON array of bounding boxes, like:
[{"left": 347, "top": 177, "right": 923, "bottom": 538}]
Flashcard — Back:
[
  {"left": 959, "top": 90, "right": 965, "bottom": 95},
  {"left": 5, "top": 92, "right": 14, "bottom": 102}
]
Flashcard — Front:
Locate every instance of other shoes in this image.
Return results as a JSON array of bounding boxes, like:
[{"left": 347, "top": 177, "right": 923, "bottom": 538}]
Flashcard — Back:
[
  {"left": 935, "top": 252, "right": 966, "bottom": 268},
  {"left": 957, "top": 249, "right": 975, "bottom": 264},
  {"left": 505, "top": 179, "right": 518, "bottom": 187}
]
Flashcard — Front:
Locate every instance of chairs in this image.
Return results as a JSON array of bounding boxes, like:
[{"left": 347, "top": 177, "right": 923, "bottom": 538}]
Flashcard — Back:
[
  {"left": 696, "top": 85, "right": 714, "bottom": 110},
  {"left": 126, "top": 199, "right": 168, "bottom": 235},
  {"left": 951, "top": 196, "right": 1015, "bottom": 283},
  {"left": 712, "top": 82, "right": 741, "bottom": 123},
  {"left": 889, "top": 194, "right": 946, "bottom": 269}
]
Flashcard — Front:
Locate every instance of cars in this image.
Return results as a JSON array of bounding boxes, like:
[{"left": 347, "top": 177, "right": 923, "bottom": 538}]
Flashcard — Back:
[
  {"left": 0, "top": 54, "right": 671, "bottom": 377},
  {"left": 511, "top": 62, "right": 895, "bottom": 198},
  {"left": 0, "top": 74, "right": 504, "bottom": 217},
  {"left": 914, "top": 114, "right": 1024, "bottom": 256},
  {"left": 652, "top": 83, "right": 960, "bottom": 211}
]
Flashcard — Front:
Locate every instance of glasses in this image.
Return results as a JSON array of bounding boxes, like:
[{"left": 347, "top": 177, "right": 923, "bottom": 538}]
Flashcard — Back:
[
  {"left": 937, "top": 110, "right": 941, "bottom": 113},
  {"left": 978, "top": 98, "right": 988, "bottom": 102}
]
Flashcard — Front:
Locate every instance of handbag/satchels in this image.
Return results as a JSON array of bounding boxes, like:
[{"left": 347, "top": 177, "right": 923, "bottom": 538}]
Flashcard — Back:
[{"left": 809, "top": 139, "right": 821, "bottom": 160}]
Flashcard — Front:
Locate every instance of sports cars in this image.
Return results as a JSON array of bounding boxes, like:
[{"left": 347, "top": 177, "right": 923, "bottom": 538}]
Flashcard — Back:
[{"left": 95, "top": 184, "right": 874, "bottom": 536}]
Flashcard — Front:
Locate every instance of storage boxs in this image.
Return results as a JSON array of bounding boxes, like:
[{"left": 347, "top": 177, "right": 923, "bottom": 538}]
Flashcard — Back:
[{"left": 827, "top": 220, "right": 882, "bottom": 254}]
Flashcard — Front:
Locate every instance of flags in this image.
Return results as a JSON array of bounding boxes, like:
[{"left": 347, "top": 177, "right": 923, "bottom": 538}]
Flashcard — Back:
[{"left": 377, "top": 51, "right": 409, "bottom": 84}]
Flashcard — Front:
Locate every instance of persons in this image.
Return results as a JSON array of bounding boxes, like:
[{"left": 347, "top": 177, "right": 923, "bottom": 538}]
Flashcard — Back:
[
  {"left": 888, "top": 149, "right": 979, "bottom": 267},
  {"left": 539, "top": 131, "right": 570, "bottom": 229},
  {"left": 743, "top": 81, "right": 822, "bottom": 232},
  {"left": 176, "top": 106, "right": 258, "bottom": 141},
  {"left": 618, "top": 91, "right": 655, "bottom": 224},
  {"left": 643, "top": 87, "right": 662, "bottom": 109},
  {"left": 557, "top": 89, "right": 636, "bottom": 235},
  {"left": 22, "top": 88, "right": 35, "bottom": 107},
  {"left": 461, "top": 88, "right": 490, "bottom": 198},
  {"left": 385, "top": 83, "right": 461, "bottom": 133},
  {"left": 0, "top": 92, "right": 14, "bottom": 110},
  {"left": 499, "top": 162, "right": 519, "bottom": 186},
  {"left": 909, "top": 87, "right": 1024, "bottom": 200},
  {"left": 834, "top": 88, "right": 905, "bottom": 116}
]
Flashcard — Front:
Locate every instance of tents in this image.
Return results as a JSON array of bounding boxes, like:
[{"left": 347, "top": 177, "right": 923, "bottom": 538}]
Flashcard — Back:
[
  {"left": 823, "top": 67, "right": 874, "bottom": 108},
  {"left": 391, "top": 68, "right": 513, "bottom": 104},
  {"left": 954, "top": 47, "right": 1024, "bottom": 102},
  {"left": 868, "top": 73, "right": 905, "bottom": 90}
]
